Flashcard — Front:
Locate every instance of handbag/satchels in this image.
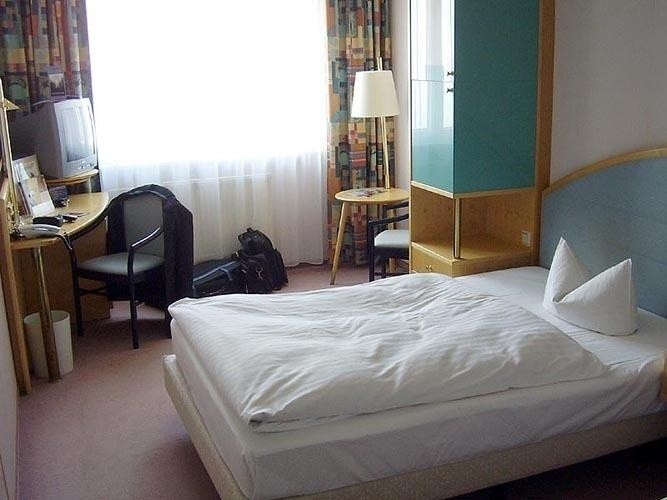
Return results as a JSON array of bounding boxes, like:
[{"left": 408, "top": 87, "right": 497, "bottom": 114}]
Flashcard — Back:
[{"left": 192, "top": 228, "right": 288, "bottom": 298}]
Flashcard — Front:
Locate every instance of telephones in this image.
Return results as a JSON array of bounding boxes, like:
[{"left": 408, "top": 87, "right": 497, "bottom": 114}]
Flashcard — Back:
[{"left": 20, "top": 224, "right": 61, "bottom": 239}]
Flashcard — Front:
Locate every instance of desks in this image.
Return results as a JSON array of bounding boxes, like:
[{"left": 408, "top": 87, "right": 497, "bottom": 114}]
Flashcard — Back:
[
  {"left": 11, "top": 191, "right": 111, "bottom": 383},
  {"left": 331, "top": 187, "right": 409, "bottom": 284}
]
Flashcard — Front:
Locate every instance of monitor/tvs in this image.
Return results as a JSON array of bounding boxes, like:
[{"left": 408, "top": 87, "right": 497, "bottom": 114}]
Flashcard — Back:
[{"left": 8, "top": 98, "right": 97, "bottom": 178}]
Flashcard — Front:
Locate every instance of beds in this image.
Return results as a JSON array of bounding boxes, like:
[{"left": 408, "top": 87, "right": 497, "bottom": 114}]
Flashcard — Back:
[{"left": 162, "top": 150, "right": 667, "bottom": 499}]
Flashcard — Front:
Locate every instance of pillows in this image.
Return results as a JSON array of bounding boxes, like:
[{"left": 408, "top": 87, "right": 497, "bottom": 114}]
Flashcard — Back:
[{"left": 542, "top": 235, "right": 640, "bottom": 337}]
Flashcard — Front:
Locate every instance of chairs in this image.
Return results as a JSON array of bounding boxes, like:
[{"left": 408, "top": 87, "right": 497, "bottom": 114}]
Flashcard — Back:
[
  {"left": 66, "top": 184, "right": 178, "bottom": 349},
  {"left": 367, "top": 202, "right": 410, "bottom": 282}
]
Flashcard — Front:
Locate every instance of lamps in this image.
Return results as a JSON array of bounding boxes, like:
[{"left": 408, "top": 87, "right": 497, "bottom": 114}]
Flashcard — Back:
[{"left": 350, "top": 56, "right": 401, "bottom": 272}]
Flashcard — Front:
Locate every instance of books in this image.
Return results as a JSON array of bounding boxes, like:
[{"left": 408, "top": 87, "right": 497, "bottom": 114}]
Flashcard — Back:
[{"left": 10, "top": 153, "right": 56, "bottom": 218}]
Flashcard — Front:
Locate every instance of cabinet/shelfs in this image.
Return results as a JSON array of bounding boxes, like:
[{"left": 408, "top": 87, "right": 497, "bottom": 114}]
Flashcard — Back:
[{"left": 408, "top": 1, "right": 555, "bottom": 278}]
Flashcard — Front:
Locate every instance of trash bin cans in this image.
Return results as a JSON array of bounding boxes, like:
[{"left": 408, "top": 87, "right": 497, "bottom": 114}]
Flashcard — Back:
[{"left": 23, "top": 311, "right": 74, "bottom": 379}]
